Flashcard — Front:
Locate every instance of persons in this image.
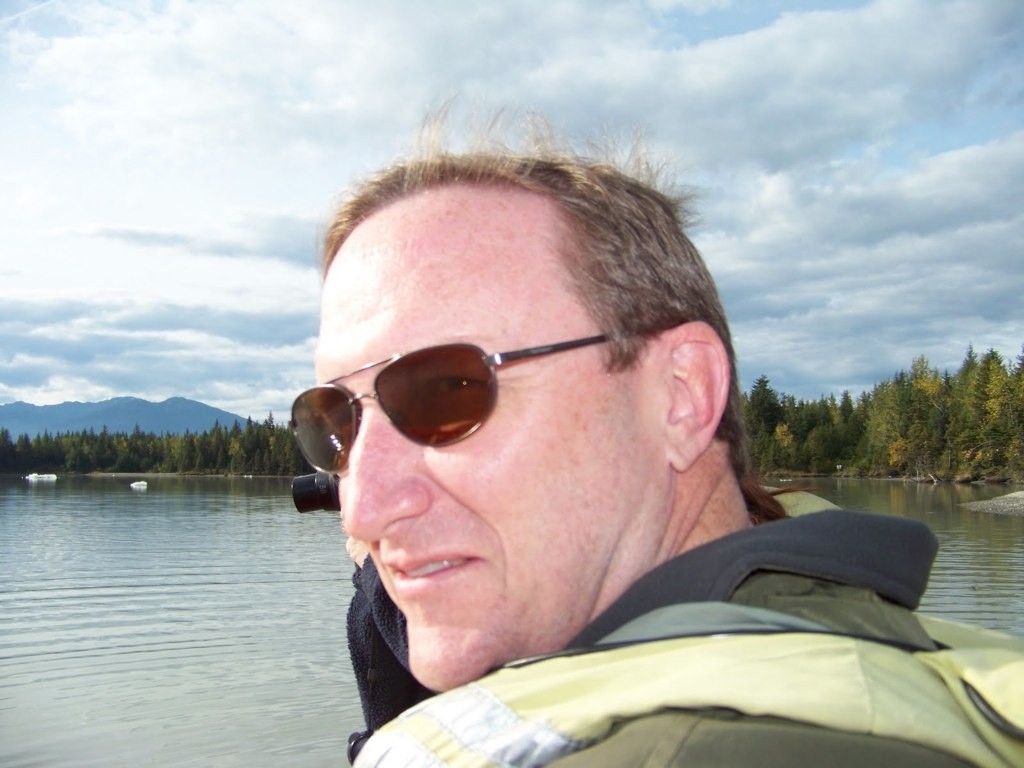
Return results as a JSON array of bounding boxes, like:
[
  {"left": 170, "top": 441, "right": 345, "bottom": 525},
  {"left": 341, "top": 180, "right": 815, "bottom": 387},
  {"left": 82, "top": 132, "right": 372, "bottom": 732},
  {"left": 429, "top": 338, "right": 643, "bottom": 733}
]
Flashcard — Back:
[{"left": 289, "top": 154, "right": 1024, "bottom": 768}]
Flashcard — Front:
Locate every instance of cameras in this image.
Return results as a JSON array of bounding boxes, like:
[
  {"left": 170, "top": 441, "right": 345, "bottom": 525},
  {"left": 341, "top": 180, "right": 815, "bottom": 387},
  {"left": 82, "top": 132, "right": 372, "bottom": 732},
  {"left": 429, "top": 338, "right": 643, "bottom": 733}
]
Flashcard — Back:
[{"left": 291, "top": 470, "right": 342, "bottom": 513}]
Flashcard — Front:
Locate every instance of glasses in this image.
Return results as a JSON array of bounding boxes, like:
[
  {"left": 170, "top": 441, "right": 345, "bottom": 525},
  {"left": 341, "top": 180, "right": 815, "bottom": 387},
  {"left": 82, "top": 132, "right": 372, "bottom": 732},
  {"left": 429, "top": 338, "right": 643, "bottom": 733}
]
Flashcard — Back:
[{"left": 289, "top": 318, "right": 693, "bottom": 475}]
